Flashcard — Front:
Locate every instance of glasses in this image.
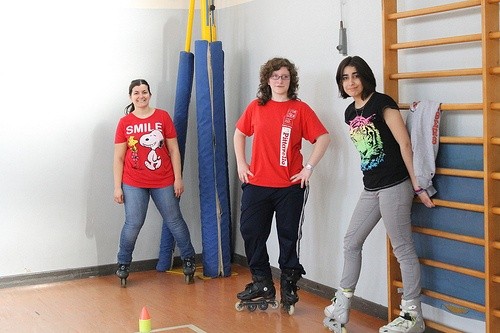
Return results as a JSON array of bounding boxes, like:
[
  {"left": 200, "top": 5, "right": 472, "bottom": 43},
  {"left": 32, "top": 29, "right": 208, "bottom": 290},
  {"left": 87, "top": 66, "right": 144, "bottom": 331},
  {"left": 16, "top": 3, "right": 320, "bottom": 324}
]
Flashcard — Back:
[{"left": 269, "top": 74, "right": 290, "bottom": 81}]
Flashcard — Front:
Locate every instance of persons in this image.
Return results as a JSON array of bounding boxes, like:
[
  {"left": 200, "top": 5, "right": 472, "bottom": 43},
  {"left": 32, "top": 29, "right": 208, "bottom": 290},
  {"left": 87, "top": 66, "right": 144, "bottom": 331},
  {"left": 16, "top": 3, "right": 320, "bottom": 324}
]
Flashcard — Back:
[
  {"left": 233, "top": 58, "right": 330, "bottom": 316},
  {"left": 324, "top": 56, "right": 435, "bottom": 333},
  {"left": 113, "top": 79, "right": 198, "bottom": 289}
]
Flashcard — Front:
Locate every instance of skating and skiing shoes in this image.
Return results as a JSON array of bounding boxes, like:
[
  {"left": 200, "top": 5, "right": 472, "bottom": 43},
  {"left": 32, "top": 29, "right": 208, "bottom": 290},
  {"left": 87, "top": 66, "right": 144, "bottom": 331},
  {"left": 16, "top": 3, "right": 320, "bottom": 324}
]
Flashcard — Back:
[
  {"left": 115, "top": 261, "right": 131, "bottom": 288},
  {"left": 235, "top": 267, "right": 279, "bottom": 312},
  {"left": 278, "top": 267, "right": 303, "bottom": 316},
  {"left": 323, "top": 286, "right": 354, "bottom": 333},
  {"left": 378, "top": 297, "right": 425, "bottom": 333},
  {"left": 183, "top": 256, "right": 196, "bottom": 284}
]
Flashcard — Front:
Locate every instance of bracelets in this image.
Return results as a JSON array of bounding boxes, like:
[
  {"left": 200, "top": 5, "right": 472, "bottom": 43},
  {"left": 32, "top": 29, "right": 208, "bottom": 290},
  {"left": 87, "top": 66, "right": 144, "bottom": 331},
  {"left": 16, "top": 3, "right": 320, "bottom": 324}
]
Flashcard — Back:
[
  {"left": 415, "top": 188, "right": 426, "bottom": 195},
  {"left": 306, "top": 163, "right": 314, "bottom": 171}
]
